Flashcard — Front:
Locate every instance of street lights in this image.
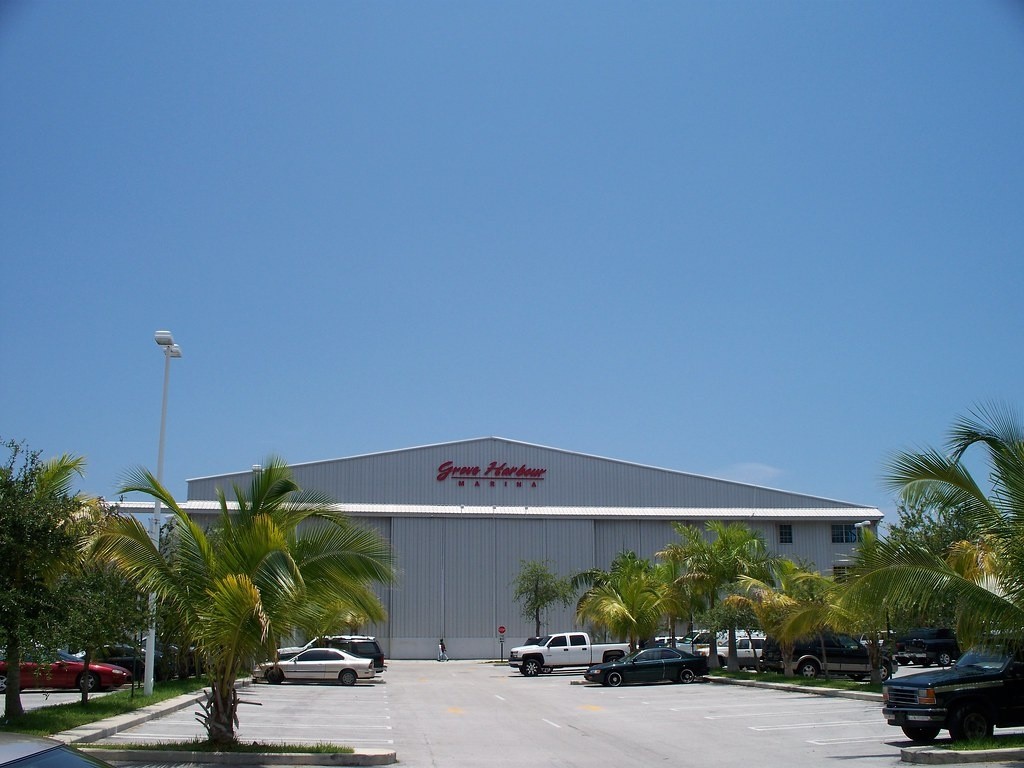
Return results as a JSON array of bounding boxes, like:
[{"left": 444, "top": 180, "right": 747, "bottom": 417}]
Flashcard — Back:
[
  {"left": 855, "top": 521, "right": 872, "bottom": 569},
  {"left": 139, "top": 331, "right": 183, "bottom": 701}
]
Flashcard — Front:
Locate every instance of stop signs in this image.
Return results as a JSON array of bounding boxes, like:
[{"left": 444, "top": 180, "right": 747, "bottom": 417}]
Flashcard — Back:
[{"left": 498, "top": 626, "right": 506, "bottom": 634}]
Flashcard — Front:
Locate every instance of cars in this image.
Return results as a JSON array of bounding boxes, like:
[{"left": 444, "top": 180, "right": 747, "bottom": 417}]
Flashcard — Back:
[
  {"left": 584, "top": 647, "right": 707, "bottom": 687},
  {"left": 253, "top": 647, "right": 377, "bottom": 686},
  {"left": 524, "top": 636, "right": 553, "bottom": 674},
  {"left": 0, "top": 643, "right": 133, "bottom": 695},
  {"left": 70, "top": 644, "right": 243, "bottom": 684}
]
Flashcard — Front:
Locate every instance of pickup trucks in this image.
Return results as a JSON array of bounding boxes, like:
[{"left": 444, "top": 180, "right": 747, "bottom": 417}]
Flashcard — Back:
[{"left": 508, "top": 632, "right": 631, "bottom": 677}]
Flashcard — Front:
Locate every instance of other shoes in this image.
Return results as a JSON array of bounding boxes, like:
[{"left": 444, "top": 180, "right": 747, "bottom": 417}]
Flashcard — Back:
[
  {"left": 447, "top": 659, "right": 449, "bottom": 661},
  {"left": 437, "top": 660, "right": 440, "bottom": 661}
]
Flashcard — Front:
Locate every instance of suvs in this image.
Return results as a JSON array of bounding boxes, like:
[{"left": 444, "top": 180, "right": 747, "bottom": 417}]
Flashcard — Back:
[
  {"left": 654, "top": 628, "right": 768, "bottom": 669},
  {"left": 276, "top": 635, "right": 387, "bottom": 674},
  {"left": 759, "top": 630, "right": 898, "bottom": 682},
  {"left": 859, "top": 627, "right": 964, "bottom": 667},
  {"left": 880, "top": 637, "right": 1024, "bottom": 743}
]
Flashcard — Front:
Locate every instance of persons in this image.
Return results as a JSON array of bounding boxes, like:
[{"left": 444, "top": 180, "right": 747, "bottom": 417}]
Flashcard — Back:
[{"left": 437, "top": 638, "right": 450, "bottom": 662}]
